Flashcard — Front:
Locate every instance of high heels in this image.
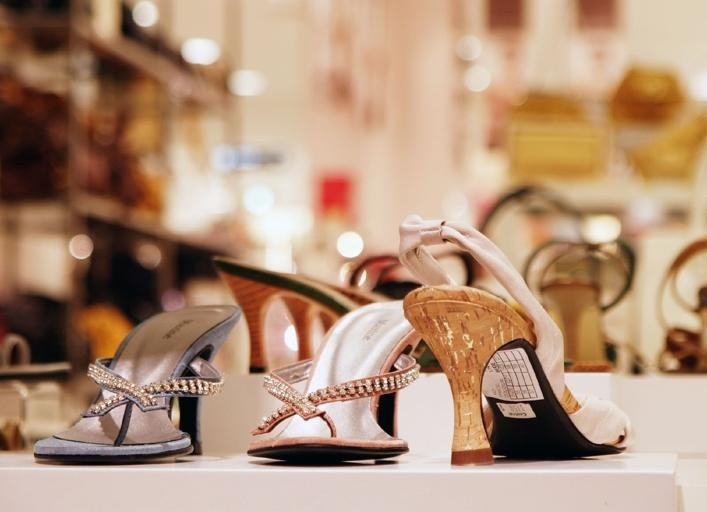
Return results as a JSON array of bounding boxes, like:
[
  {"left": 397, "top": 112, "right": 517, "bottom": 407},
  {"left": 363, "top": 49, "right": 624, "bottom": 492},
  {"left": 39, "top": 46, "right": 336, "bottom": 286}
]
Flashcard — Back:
[
  {"left": 35, "top": 219, "right": 707, "bottom": 386},
  {"left": 33, "top": 304, "right": 241, "bottom": 464},
  {"left": 398, "top": 216, "right": 635, "bottom": 464},
  {"left": 246, "top": 301, "right": 427, "bottom": 463}
]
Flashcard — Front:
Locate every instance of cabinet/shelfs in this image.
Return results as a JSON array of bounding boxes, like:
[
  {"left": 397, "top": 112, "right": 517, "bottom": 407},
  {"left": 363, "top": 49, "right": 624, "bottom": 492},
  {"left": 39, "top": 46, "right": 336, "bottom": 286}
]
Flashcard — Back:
[{"left": 1, "top": 0, "right": 246, "bottom": 380}]
